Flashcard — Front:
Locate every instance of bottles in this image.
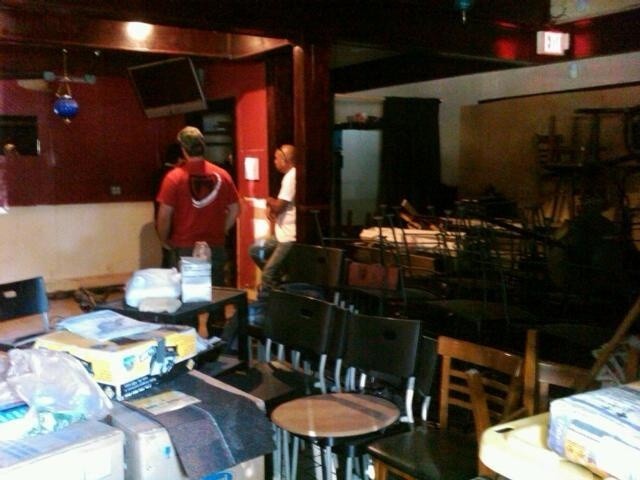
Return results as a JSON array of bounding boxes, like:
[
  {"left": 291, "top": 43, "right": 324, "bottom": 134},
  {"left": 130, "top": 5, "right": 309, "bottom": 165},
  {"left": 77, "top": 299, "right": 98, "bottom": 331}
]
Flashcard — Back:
[{"left": 191, "top": 241, "right": 212, "bottom": 261}]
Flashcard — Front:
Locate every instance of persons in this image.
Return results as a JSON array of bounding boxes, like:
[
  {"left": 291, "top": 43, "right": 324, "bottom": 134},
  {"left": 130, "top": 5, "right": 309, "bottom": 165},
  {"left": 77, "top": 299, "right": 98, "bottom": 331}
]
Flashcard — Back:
[
  {"left": 155, "top": 127, "right": 243, "bottom": 336},
  {"left": 152, "top": 138, "right": 186, "bottom": 226},
  {"left": 259, "top": 141, "right": 299, "bottom": 287}
]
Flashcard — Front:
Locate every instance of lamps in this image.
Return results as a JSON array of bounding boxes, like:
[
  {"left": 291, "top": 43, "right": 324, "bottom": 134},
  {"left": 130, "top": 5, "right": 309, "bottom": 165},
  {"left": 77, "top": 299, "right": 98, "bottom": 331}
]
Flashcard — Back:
[{"left": 53, "top": 48, "right": 80, "bottom": 125}]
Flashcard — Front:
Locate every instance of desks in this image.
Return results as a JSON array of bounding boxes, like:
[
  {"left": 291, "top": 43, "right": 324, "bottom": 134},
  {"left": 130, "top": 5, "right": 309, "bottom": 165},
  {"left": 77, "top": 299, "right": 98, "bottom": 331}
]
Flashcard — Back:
[{"left": 95, "top": 286, "right": 248, "bottom": 380}]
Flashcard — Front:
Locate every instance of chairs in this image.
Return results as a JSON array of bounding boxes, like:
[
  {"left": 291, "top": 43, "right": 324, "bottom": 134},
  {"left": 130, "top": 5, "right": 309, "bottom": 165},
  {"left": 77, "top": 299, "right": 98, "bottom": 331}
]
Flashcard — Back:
[
  {"left": 1, "top": 277, "right": 51, "bottom": 349},
  {"left": 211, "top": 206, "right": 640, "bottom": 480}
]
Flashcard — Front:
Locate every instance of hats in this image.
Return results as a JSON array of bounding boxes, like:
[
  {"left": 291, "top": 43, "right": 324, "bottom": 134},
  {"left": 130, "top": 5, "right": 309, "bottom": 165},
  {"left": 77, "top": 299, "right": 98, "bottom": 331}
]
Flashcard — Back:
[{"left": 175, "top": 124, "right": 207, "bottom": 153}]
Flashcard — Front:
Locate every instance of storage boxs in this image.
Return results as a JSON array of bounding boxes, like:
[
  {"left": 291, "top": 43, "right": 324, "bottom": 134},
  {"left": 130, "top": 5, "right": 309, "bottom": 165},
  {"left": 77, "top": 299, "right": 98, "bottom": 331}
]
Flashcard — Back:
[{"left": 0, "top": 319, "right": 267, "bottom": 480}]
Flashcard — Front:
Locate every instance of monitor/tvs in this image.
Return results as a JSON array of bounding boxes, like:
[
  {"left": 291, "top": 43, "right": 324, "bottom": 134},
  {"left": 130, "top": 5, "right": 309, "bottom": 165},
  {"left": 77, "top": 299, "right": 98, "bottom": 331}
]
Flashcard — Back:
[{"left": 127, "top": 56, "right": 207, "bottom": 120}]
